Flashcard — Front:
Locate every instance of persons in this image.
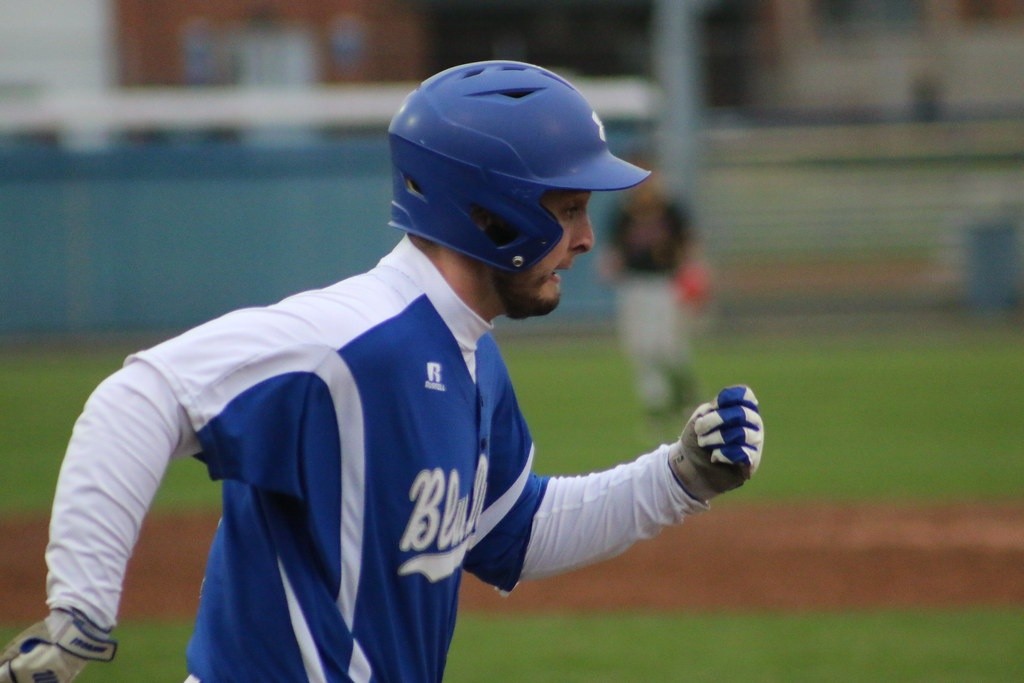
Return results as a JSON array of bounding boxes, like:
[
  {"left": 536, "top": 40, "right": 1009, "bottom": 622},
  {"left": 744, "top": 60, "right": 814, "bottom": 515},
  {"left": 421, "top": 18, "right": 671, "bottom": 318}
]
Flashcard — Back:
[
  {"left": 0, "top": 60, "right": 766, "bottom": 683},
  {"left": 598, "top": 152, "right": 710, "bottom": 427}
]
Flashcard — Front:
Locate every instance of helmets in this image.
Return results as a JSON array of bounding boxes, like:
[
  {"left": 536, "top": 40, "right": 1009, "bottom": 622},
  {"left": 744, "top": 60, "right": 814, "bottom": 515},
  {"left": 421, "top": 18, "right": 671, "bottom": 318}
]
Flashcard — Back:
[{"left": 386, "top": 60, "right": 652, "bottom": 272}]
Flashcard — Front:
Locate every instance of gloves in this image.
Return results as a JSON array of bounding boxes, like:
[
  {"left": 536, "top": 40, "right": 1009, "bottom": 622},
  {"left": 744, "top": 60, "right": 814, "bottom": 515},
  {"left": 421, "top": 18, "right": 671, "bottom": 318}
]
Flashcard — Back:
[
  {"left": 0, "top": 606, "right": 119, "bottom": 683},
  {"left": 670, "top": 385, "right": 766, "bottom": 504}
]
